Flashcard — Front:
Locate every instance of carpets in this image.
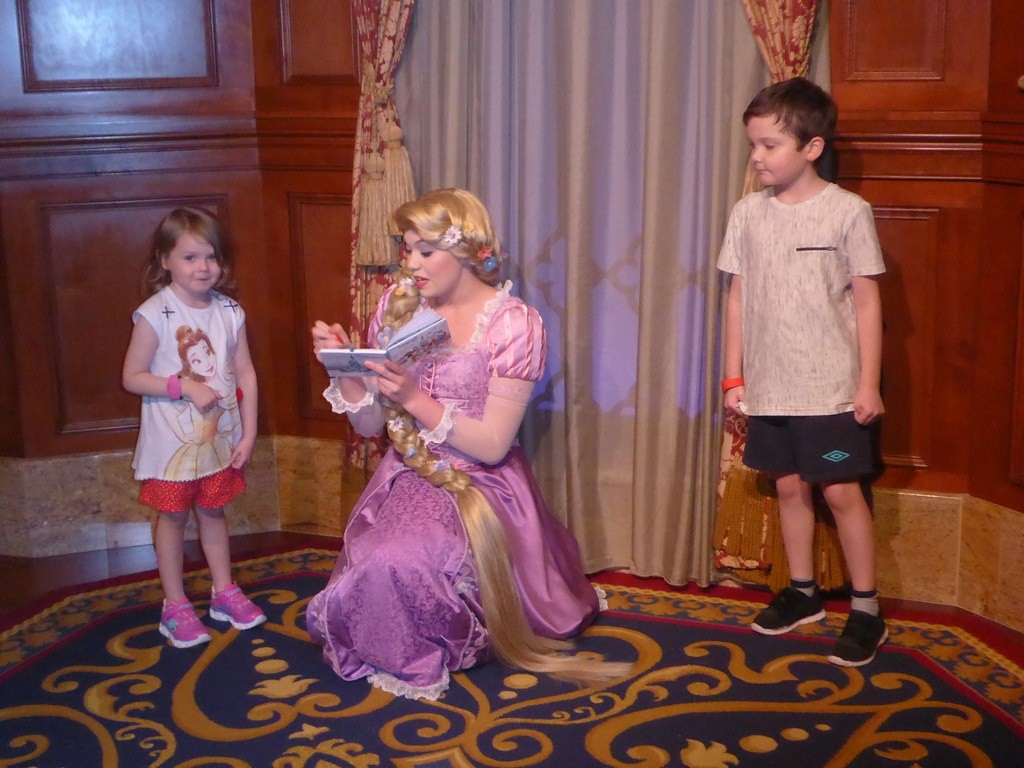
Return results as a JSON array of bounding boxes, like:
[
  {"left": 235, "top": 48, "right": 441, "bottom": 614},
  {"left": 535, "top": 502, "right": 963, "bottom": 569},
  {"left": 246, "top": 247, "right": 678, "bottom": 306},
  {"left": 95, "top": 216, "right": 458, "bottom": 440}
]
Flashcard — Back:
[{"left": 1, "top": 549, "right": 1024, "bottom": 768}]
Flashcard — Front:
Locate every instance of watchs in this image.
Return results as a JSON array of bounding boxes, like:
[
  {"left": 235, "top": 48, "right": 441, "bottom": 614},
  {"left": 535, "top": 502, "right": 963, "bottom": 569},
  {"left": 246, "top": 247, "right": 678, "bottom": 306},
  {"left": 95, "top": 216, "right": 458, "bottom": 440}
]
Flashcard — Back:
[{"left": 168, "top": 375, "right": 182, "bottom": 401}]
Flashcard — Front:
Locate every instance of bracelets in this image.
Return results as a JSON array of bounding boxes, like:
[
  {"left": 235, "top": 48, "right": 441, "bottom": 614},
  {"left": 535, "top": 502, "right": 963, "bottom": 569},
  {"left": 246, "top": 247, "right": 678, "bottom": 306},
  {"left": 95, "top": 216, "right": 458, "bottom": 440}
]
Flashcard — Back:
[{"left": 721, "top": 377, "right": 743, "bottom": 393}]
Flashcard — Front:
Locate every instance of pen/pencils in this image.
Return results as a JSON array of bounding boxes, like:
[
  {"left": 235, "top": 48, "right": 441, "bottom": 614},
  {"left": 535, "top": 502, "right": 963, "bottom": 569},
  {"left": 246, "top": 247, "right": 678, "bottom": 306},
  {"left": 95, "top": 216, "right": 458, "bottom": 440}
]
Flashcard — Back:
[{"left": 335, "top": 333, "right": 345, "bottom": 345}]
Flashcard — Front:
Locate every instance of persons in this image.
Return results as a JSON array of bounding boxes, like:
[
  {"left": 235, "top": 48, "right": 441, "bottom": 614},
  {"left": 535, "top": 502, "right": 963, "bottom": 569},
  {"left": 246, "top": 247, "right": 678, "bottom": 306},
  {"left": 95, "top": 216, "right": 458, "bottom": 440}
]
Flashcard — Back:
[
  {"left": 715, "top": 77, "right": 892, "bottom": 668},
  {"left": 305, "top": 187, "right": 633, "bottom": 700},
  {"left": 121, "top": 204, "right": 269, "bottom": 650}
]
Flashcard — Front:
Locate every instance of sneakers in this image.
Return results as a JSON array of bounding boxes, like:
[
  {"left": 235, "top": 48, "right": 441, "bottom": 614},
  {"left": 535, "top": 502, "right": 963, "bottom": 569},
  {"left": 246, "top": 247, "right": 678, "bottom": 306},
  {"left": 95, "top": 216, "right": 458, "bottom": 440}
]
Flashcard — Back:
[
  {"left": 209, "top": 581, "right": 268, "bottom": 631},
  {"left": 159, "top": 599, "right": 212, "bottom": 648},
  {"left": 751, "top": 586, "right": 826, "bottom": 634},
  {"left": 828, "top": 609, "right": 888, "bottom": 666}
]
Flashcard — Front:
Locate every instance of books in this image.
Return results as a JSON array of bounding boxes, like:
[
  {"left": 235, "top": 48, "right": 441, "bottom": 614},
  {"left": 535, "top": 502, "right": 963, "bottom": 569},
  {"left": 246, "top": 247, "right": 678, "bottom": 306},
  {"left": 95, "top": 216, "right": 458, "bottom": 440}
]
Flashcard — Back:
[{"left": 319, "top": 305, "right": 451, "bottom": 378}]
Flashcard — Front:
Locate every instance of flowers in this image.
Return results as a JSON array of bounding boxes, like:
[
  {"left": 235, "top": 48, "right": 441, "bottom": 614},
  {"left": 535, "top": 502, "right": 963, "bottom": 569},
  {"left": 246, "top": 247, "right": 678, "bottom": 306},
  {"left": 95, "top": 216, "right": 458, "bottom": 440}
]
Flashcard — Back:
[
  {"left": 443, "top": 226, "right": 462, "bottom": 244},
  {"left": 499, "top": 247, "right": 510, "bottom": 262},
  {"left": 477, "top": 245, "right": 493, "bottom": 259},
  {"left": 376, "top": 277, "right": 450, "bottom": 471}
]
